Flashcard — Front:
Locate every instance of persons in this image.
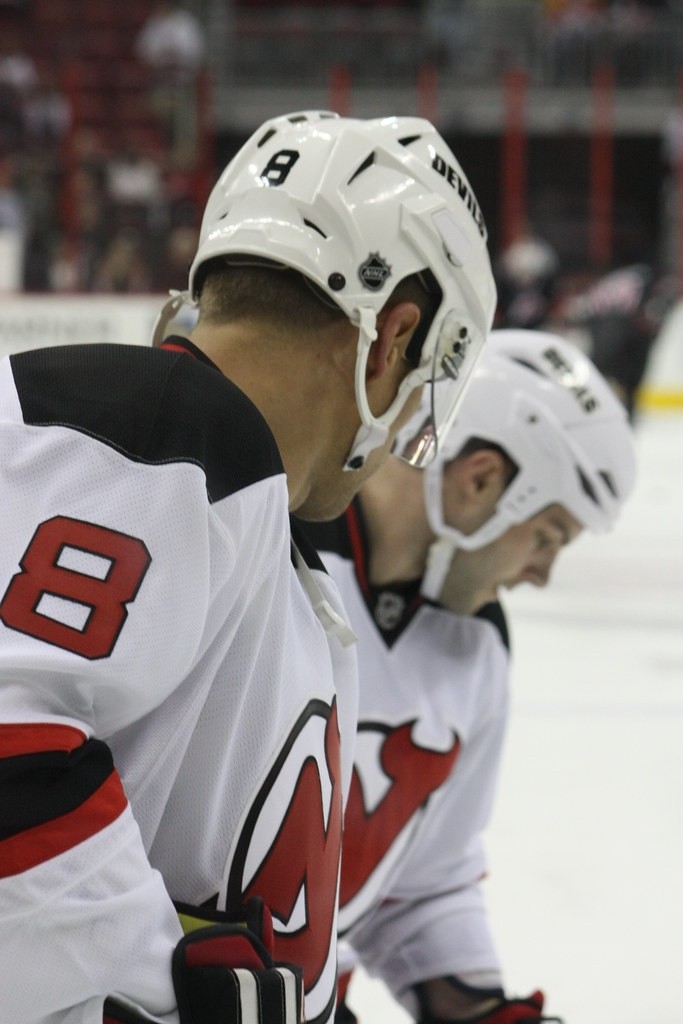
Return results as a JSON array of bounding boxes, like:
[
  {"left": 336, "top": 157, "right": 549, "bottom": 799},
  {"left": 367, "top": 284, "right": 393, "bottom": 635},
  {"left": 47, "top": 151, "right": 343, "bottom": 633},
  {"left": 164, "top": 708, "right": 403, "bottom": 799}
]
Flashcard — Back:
[
  {"left": 0, "top": 0, "right": 682, "bottom": 429},
  {"left": 293, "top": 324, "right": 642, "bottom": 1024},
  {"left": 0, "top": 110, "right": 500, "bottom": 1024}
]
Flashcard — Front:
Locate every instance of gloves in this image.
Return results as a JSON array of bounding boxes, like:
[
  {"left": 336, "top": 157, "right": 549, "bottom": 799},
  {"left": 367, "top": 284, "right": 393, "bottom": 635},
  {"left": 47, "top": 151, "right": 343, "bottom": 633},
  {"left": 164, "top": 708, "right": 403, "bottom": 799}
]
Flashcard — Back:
[
  {"left": 413, "top": 982, "right": 562, "bottom": 1024},
  {"left": 103, "top": 895, "right": 306, "bottom": 1024}
]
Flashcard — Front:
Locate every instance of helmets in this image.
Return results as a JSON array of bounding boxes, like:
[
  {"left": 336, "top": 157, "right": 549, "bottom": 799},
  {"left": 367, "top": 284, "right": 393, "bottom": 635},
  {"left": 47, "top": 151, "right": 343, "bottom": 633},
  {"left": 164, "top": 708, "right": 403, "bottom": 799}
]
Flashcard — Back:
[
  {"left": 152, "top": 109, "right": 498, "bottom": 472},
  {"left": 422, "top": 327, "right": 640, "bottom": 551}
]
